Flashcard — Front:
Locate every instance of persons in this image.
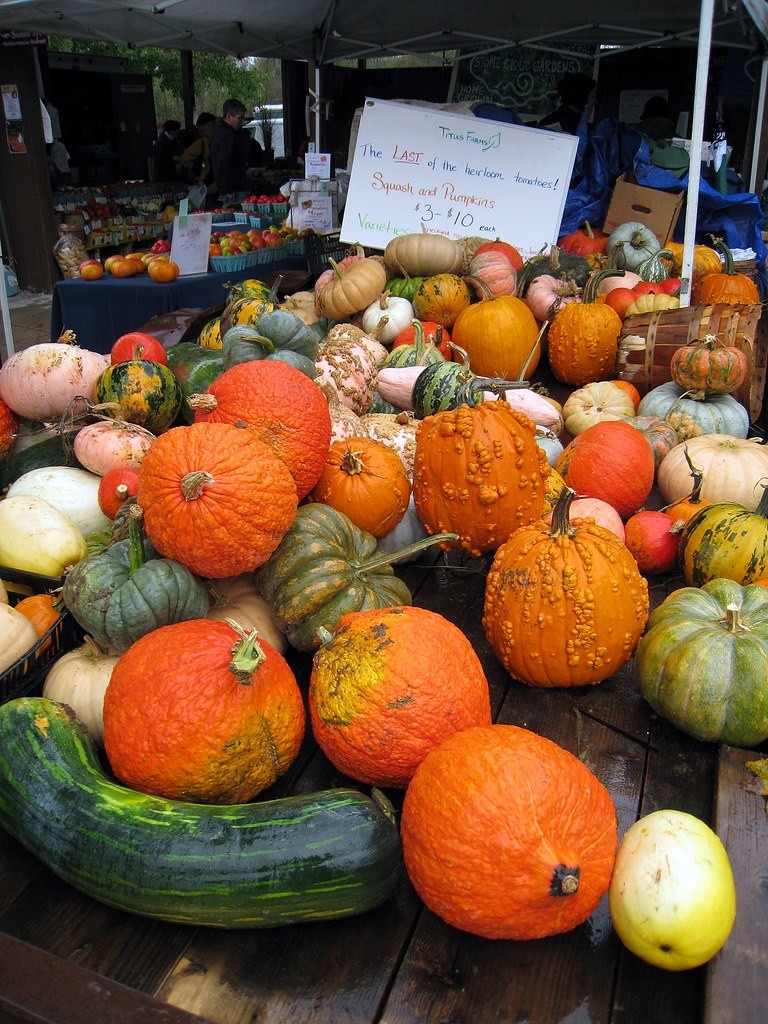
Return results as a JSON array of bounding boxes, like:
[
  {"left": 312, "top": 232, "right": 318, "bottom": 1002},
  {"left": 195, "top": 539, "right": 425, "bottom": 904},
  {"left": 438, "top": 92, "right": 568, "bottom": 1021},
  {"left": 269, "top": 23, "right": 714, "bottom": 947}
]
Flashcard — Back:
[
  {"left": 47, "top": 99, "right": 308, "bottom": 212},
  {"left": 537, "top": 72, "right": 598, "bottom": 136},
  {"left": 636, "top": 94, "right": 679, "bottom": 140}
]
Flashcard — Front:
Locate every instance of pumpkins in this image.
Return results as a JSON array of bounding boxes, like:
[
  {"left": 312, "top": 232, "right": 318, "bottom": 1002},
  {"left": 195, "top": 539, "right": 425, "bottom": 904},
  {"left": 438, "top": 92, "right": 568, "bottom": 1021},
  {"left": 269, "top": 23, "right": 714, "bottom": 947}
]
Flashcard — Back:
[{"left": 0, "top": 222, "right": 768, "bottom": 968}]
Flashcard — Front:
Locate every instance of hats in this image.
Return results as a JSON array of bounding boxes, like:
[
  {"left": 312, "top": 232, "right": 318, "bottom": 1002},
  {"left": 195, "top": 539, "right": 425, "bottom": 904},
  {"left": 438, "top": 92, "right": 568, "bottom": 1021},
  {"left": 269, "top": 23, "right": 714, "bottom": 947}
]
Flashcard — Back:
[{"left": 197, "top": 113, "right": 216, "bottom": 125}]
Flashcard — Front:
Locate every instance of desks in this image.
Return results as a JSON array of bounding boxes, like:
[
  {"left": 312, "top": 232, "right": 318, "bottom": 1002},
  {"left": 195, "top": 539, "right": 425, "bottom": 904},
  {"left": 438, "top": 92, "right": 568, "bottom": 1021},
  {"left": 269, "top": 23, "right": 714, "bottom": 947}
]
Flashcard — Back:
[
  {"left": 50, "top": 253, "right": 310, "bottom": 356},
  {"left": 0, "top": 544, "right": 767, "bottom": 1023}
]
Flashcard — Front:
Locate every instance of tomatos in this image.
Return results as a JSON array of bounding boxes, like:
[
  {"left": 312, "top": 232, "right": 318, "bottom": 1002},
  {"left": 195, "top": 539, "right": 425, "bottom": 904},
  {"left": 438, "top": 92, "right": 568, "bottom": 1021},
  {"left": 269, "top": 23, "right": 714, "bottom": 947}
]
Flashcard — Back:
[{"left": 79, "top": 196, "right": 315, "bottom": 283}]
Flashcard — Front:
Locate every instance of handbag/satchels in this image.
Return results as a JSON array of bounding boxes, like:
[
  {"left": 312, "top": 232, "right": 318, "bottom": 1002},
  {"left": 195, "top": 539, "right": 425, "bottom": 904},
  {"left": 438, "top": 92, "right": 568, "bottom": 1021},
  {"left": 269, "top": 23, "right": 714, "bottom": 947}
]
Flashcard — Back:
[{"left": 192, "top": 139, "right": 205, "bottom": 176}]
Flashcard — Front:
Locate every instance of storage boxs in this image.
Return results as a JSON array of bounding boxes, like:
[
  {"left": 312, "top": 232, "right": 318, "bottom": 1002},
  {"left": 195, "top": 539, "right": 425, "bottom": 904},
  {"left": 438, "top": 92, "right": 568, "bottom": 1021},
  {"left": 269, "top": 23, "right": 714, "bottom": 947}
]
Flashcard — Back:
[
  {"left": 603, "top": 171, "right": 684, "bottom": 249},
  {"left": 0, "top": 564, "right": 91, "bottom": 707},
  {"left": 287, "top": 230, "right": 356, "bottom": 271}
]
